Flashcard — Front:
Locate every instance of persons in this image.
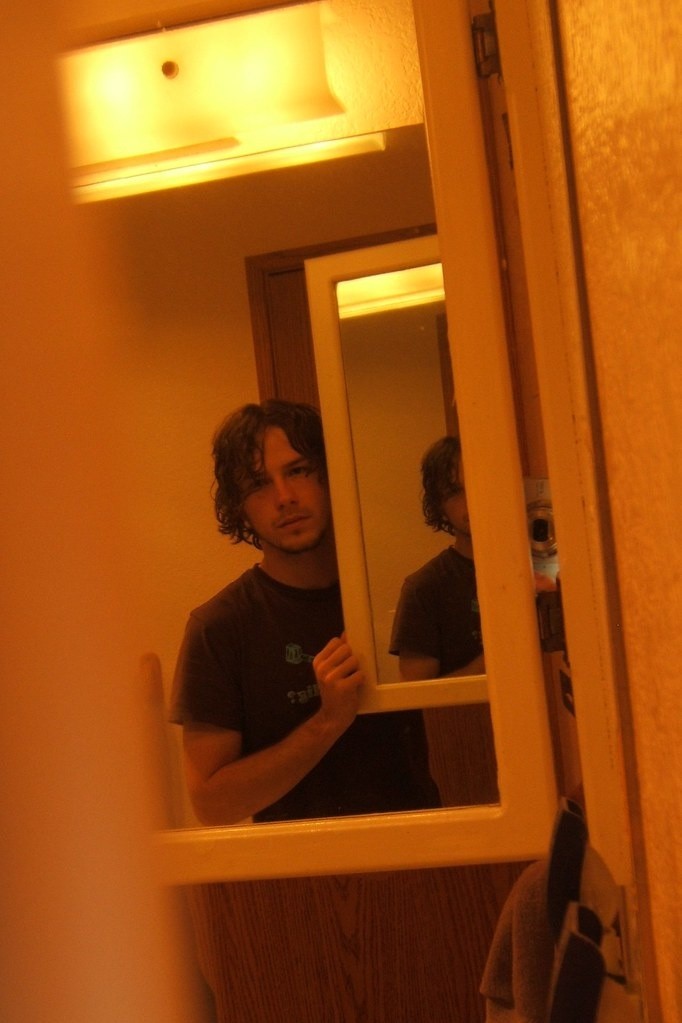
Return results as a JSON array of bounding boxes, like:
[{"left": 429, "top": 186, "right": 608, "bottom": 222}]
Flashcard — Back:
[
  {"left": 184, "top": 399, "right": 442, "bottom": 827},
  {"left": 387, "top": 436, "right": 486, "bottom": 682}
]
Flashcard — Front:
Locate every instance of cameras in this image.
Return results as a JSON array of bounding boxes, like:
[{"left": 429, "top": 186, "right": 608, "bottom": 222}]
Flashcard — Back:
[{"left": 523, "top": 475, "right": 560, "bottom": 594}]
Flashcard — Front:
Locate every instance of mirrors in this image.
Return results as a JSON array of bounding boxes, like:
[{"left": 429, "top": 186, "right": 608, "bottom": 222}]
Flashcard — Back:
[
  {"left": 302, "top": 232, "right": 489, "bottom": 716},
  {"left": 0, "top": 2, "right": 557, "bottom": 884}
]
